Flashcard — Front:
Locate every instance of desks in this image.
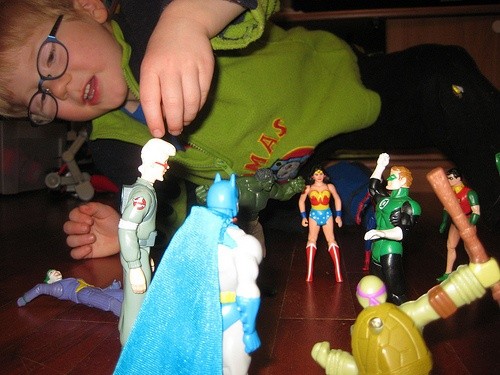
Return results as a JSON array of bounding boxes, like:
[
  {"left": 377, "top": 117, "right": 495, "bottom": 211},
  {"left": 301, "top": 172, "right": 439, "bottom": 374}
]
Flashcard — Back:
[{"left": 270, "top": 3, "right": 500, "bottom": 197}]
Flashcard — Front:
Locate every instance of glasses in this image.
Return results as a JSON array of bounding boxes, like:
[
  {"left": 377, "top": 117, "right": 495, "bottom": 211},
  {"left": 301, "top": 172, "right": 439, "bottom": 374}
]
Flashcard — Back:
[{"left": 28, "top": 15, "right": 69, "bottom": 127}]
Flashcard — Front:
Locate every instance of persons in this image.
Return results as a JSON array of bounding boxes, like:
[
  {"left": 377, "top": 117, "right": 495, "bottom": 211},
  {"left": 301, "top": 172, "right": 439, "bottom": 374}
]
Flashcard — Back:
[
  {"left": 0, "top": 0, "right": 500, "bottom": 261},
  {"left": 15, "top": 138, "right": 500, "bottom": 375}
]
